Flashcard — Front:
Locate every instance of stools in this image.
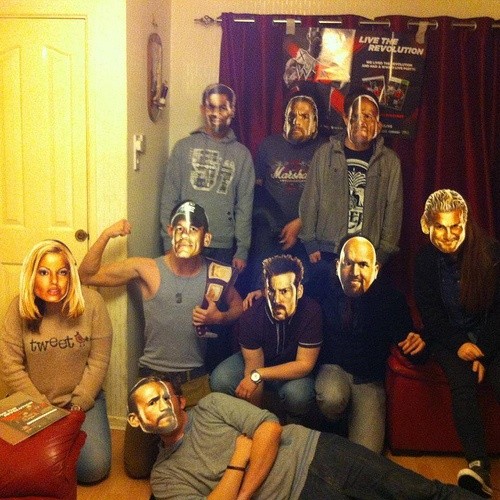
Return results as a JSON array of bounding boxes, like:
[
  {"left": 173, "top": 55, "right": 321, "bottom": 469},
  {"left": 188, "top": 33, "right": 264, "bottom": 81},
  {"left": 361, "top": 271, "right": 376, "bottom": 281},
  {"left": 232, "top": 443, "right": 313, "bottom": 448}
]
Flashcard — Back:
[{"left": 385, "top": 342, "right": 500, "bottom": 453}]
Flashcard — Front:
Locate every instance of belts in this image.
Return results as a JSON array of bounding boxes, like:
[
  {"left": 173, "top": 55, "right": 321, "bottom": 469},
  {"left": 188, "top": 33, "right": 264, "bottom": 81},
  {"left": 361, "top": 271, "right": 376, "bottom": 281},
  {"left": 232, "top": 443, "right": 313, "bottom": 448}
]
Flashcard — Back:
[{"left": 139, "top": 365, "right": 208, "bottom": 383}]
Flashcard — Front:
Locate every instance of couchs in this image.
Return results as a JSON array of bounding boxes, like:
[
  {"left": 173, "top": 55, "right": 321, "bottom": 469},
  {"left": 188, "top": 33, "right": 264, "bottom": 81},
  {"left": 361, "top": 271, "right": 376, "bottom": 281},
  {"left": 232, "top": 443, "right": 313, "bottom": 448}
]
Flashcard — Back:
[{"left": 0, "top": 408, "right": 88, "bottom": 500}]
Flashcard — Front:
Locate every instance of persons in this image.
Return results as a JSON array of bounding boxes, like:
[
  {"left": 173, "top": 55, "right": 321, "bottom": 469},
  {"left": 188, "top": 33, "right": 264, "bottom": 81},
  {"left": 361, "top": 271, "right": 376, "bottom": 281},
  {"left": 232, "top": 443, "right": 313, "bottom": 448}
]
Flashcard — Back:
[
  {"left": 297, "top": 88, "right": 404, "bottom": 273},
  {"left": 78, "top": 200, "right": 245, "bottom": 480},
  {"left": 1, "top": 239, "right": 114, "bottom": 485},
  {"left": 159, "top": 84, "right": 255, "bottom": 287},
  {"left": 409, "top": 190, "right": 500, "bottom": 496},
  {"left": 255, "top": 94, "right": 327, "bottom": 288},
  {"left": 127, "top": 377, "right": 488, "bottom": 500},
  {"left": 241, "top": 234, "right": 425, "bottom": 456},
  {"left": 209, "top": 256, "right": 324, "bottom": 430}
]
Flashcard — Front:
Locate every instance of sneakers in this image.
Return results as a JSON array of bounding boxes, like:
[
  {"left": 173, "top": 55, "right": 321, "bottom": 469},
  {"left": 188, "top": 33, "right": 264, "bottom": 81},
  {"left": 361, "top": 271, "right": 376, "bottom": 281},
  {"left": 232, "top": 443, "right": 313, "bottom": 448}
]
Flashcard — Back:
[{"left": 458, "top": 469, "right": 494, "bottom": 497}]
